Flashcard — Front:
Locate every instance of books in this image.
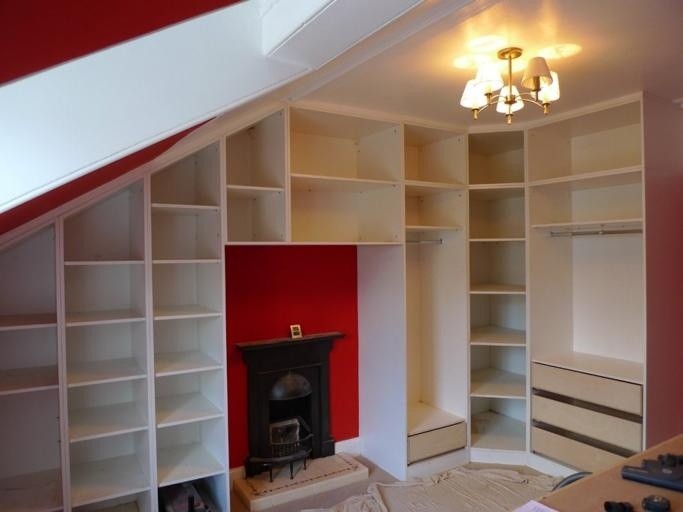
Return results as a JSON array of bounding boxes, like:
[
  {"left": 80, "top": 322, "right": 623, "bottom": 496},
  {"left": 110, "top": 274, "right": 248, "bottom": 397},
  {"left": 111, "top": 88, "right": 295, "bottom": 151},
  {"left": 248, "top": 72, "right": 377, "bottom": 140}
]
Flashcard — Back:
[{"left": 160, "top": 482, "right": 205, "bottom": 511}]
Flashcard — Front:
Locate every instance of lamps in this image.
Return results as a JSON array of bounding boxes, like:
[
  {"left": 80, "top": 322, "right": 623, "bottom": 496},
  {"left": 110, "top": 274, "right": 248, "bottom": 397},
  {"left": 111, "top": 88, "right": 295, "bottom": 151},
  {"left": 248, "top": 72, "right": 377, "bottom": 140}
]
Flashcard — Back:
[{"left": 451, "top": 33, "right": 582, "bottom": 126}]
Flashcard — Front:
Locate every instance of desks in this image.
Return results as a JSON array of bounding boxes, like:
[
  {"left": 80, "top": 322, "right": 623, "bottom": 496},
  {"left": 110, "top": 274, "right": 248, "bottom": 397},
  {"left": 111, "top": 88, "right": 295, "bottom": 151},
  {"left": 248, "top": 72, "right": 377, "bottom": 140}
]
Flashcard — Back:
[{"left": 513, "top": 429, "right": 683, "bottom": 512}]
